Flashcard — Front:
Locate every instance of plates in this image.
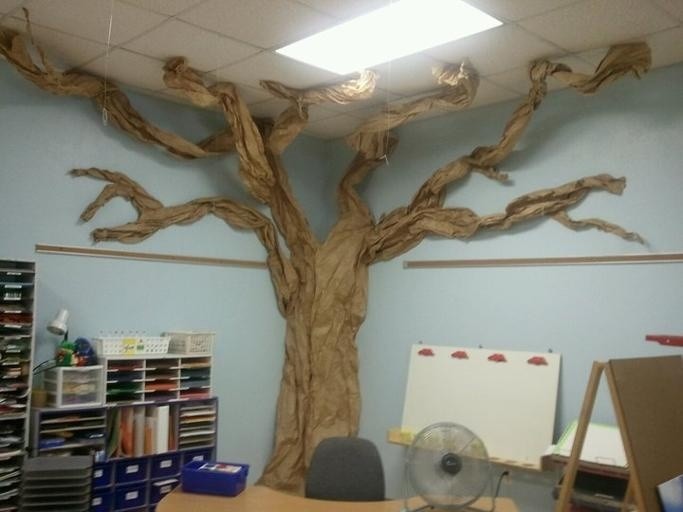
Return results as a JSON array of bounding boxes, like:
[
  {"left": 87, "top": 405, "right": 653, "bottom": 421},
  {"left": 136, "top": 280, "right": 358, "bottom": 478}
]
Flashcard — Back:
[
  {"left": 35, "top": 351, "right": 219, "bottom": 512},
  {"left": 0, "top": 259, "right": 37, "bottom": 511}
]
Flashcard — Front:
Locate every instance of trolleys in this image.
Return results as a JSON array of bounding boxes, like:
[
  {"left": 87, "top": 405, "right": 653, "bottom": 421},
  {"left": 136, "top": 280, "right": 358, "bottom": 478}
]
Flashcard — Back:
[{"left": 405, "top": 421, "right": 494, "bottom": 512}]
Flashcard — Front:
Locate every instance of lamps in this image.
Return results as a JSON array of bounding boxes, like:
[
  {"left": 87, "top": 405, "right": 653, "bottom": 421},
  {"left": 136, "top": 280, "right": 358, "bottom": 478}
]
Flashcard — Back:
[{"left": 302, "top": 436, "right": 386, "bottom": 502}]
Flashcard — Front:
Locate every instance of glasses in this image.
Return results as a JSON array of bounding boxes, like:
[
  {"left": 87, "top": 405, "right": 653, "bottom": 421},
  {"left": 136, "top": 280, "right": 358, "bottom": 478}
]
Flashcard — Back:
[{"left": 45, "top": 308, "right": 75, "bottom": 362}]
